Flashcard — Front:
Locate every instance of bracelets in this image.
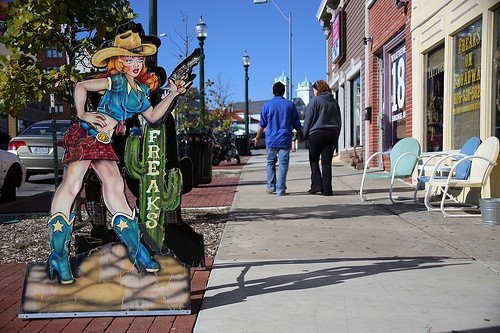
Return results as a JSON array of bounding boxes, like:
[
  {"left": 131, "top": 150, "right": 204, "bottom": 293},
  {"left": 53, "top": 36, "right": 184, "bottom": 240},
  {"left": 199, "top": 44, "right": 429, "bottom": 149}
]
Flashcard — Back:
[{"left": 256, "top": 138, "right": 260, "bottom": 139}]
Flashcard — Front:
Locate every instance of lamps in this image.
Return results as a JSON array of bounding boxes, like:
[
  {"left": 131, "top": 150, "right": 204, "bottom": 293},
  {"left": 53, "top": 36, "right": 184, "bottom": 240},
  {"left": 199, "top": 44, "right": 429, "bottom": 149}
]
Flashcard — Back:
[
  {"left": 362, "top": 36, "right": 373, "bottom": 45},
  {"left": 394, "top": 0, "right": 408, "bottom": 14}
]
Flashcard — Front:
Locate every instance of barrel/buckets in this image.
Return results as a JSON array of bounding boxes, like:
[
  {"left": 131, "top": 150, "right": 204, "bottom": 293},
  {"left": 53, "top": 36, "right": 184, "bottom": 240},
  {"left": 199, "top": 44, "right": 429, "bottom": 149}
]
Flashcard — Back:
[{"left": 479, "top": 198, "right": 500, "bottom": 226}]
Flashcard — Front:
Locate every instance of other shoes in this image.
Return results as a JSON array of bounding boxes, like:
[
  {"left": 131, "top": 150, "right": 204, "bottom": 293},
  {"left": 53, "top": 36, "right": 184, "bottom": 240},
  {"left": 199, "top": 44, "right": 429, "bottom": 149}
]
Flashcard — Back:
[
  {"left": 307, "top": 187, "right": 322, "bottom": 194},
  {"left": 323, "top": 190, "right": 334, "bottom": 196},
  {"left": 277, "top": 191, "right": 287, "bottom": 196},
  {"left": 265, "top": 187, "right": 275, "bottom": 193}
]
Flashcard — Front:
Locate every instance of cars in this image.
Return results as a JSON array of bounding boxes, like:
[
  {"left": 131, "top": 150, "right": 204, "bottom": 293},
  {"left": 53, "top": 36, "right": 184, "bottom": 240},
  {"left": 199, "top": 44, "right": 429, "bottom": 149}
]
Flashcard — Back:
[
  {"left": 0, "top": 148, "right": 28, "bottom": 205},
  {"left": 7, "top": 118, "right": 72, "bottom": 184}
]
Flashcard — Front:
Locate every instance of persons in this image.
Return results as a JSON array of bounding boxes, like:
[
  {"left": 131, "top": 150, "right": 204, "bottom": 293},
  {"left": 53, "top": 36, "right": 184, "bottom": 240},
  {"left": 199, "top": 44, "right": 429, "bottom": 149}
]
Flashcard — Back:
[
  {"left": 252, "top": 81, "right": 303, "bottom": 196},
  {"left": 303, "top": 80, "right": 342, "bottom": 195}
]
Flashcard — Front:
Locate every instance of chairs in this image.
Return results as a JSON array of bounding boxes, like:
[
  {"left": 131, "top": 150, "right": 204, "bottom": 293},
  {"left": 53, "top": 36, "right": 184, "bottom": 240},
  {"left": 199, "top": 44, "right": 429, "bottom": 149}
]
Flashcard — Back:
[
  {"left": 424, "top": 137, "right": 500, "bottom": 218},
  {"left": 413, "top": 137, "right": 483, "bottom": 208},
  {"left": 360, "top": 137, "right": 420, "bottom": 204}
]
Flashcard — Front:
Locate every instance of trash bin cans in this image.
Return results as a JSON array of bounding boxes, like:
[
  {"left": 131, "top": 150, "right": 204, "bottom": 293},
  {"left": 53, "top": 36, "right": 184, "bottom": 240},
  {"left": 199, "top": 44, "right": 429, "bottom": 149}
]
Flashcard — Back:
[
  {"left": 235, "top": 135, "right": 250, "bottom": 155},
  {"left": 177, "top": 132, "right": 213, "bottom": 184}
]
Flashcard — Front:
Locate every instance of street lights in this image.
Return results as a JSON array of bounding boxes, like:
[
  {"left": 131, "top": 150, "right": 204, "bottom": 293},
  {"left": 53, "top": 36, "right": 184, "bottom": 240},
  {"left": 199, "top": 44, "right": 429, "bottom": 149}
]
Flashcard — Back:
[
  {"left": 252, "top": 0, "right": 293, "bottom": 101},
  {"left": 240, "top": 48, "right": 252, "bottom": 136},
  {"left": 194, "top": 13, "right": 209, "bottom": 131}
]
